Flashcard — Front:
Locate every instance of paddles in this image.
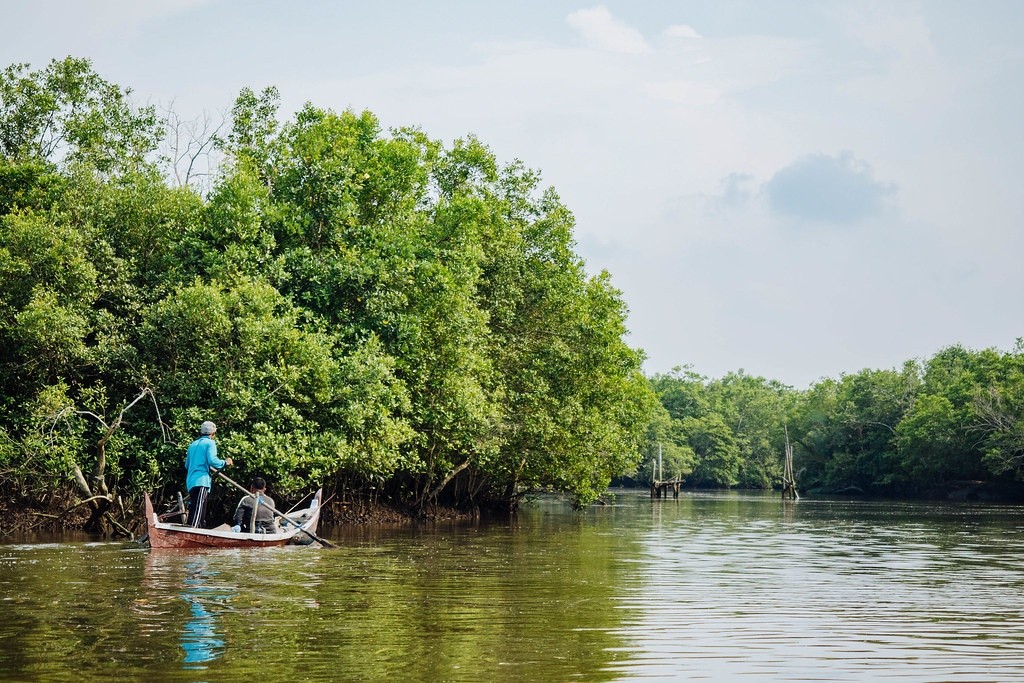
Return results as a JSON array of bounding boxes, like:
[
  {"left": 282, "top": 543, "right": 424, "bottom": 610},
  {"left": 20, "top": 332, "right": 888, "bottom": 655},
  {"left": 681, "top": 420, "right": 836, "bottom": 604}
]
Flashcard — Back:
[
  {"left": 139, "top": 462, "right": 229, "bottom": 544},
  {"left": 210, "top": 465, "right": 341, "bottom": 548}
]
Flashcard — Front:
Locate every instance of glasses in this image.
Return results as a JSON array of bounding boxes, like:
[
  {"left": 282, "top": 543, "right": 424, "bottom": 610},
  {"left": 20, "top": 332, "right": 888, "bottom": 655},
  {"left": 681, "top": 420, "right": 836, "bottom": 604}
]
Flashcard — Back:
[{"left": 215, "top": 432, "right": 217, "bottom": 434}]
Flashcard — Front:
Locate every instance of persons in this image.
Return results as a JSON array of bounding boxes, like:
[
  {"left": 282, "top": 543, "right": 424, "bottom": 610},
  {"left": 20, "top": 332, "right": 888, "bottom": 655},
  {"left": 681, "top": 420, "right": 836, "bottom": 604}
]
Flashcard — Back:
[
  {"left": 232, "top": 477, "right": 277, "bottom": 533},
  {"left": 184, "top": 421, "right": 233, "bottom": 527}
]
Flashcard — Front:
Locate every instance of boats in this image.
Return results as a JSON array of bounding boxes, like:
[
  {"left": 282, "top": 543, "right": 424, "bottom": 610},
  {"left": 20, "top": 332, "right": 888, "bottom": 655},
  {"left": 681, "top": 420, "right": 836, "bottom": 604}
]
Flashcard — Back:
[{"left": 143, "top": 485, "right": 324, "bottom": 549}]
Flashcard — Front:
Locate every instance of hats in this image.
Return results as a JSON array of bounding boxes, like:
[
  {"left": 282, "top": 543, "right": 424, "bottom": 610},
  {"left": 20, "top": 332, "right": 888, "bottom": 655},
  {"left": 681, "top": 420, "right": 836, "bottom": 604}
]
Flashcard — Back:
[
  {"left": 250, "top": 477, "right": 266, "bottom": 489},
  {"left": 201, "top": 421, "right": 217, "bottom": 434}
]
